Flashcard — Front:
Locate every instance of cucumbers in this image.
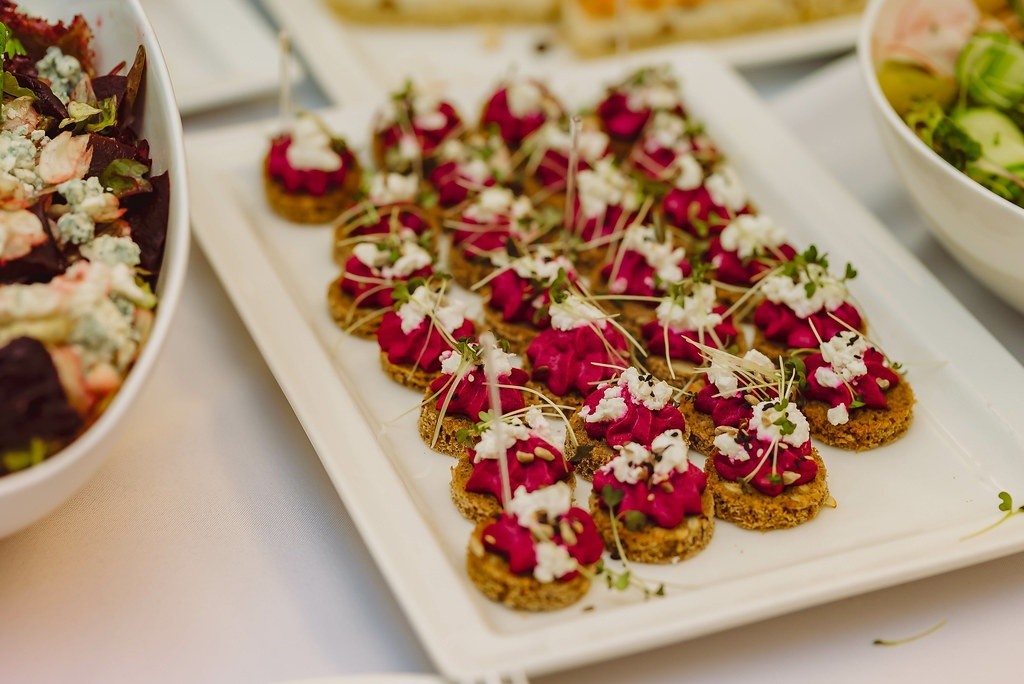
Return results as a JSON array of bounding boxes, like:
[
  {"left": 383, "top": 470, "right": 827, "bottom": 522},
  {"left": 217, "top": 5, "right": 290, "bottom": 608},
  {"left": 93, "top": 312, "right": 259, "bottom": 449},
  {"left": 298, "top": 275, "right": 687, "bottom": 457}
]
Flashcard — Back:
[{"left": 929, "top": 29, "right": 1024, "bottom": 201}]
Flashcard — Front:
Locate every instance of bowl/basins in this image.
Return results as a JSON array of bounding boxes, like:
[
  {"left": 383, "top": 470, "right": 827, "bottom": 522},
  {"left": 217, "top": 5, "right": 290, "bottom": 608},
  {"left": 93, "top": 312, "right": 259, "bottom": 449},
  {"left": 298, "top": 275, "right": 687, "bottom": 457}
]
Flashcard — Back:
[
  {"left": 0, "top": 0, "right": 188, "bottom": 536},
  {"left": 856, "top": 0, "right": 1024, "bottom": 316}
]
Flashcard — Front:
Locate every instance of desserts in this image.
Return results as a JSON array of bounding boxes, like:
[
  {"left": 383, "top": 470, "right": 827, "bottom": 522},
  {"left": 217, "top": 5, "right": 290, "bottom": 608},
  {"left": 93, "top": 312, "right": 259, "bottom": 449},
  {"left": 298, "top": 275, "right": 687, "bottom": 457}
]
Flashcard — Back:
[{"left": 261, "top": 67, "right": 917, "bottom": 615}]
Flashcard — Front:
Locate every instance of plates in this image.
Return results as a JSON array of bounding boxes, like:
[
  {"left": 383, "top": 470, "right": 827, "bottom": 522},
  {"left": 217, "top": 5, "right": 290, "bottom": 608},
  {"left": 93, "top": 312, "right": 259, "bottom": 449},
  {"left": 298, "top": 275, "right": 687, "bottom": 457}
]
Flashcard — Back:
[
  {"left": 140, "top": 0, "right": 304, "bottom": 117},
  {"left": 182, "top": 50, "right": 1024, "bottom": 683},
  {"left": 257, "top": 1, "right": 860, "bottom": 106}
]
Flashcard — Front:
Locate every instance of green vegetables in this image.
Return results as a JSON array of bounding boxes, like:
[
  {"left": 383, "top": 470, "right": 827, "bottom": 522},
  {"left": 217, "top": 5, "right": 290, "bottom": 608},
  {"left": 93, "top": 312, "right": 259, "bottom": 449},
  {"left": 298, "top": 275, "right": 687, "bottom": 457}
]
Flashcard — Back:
[{"left": 0, "top": 20, "right": 156, "bottom": 477}]
